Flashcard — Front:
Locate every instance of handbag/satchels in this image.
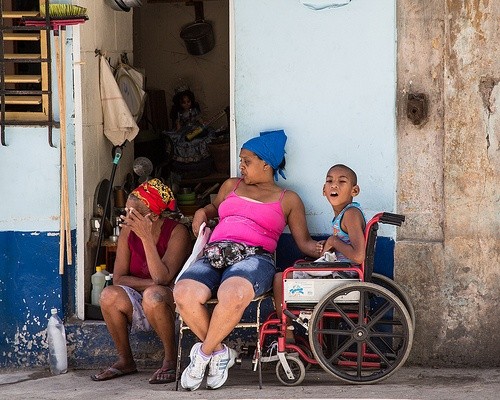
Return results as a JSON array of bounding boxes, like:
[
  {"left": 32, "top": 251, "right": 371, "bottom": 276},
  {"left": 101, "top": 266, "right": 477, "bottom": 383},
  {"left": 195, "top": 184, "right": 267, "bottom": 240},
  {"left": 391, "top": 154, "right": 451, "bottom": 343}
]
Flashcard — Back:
[{"left": 203, "top": 239, "right": 264, "bottom": 269}]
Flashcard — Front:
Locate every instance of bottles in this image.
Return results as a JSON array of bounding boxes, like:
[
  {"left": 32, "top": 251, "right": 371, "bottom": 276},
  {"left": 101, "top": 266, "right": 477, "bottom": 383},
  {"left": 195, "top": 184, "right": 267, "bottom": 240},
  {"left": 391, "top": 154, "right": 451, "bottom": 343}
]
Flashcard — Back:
[
  {"left": 47, "top": 308, "right": 68, "bottom": 376},
  {"left": 91, "top": 265, "right": 113, "bottom": 306}
]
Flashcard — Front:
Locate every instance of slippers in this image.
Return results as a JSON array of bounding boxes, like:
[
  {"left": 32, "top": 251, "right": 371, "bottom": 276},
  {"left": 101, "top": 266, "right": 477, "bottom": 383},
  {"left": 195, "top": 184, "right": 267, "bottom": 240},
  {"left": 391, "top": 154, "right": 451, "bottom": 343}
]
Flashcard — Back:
[
  {"left": 148, "top": 368, "right": 182, "bottom": 384},
  {"left": 91, "top": 365, "right": 137, "bottom": 381}
]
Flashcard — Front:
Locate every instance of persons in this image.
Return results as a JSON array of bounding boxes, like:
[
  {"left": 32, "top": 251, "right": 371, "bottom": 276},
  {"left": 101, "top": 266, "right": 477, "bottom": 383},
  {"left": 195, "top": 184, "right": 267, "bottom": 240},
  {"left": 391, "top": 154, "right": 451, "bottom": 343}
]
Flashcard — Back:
[
  {"left": 259, "top": 164, "right": 367, "bottom": 363},
  {"left": 90, "top": 178, "right": 188, "bottom": 383},
  {"left": 173, "top": 130, "right": 316, "bottom": 392}
]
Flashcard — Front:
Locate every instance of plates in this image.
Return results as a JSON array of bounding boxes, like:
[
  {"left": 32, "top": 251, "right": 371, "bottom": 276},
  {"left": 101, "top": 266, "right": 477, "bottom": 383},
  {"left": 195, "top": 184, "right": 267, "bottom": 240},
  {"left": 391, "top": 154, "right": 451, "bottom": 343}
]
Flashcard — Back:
[{"left": 178, "top": 200, "right": 195, "bottom": 205}]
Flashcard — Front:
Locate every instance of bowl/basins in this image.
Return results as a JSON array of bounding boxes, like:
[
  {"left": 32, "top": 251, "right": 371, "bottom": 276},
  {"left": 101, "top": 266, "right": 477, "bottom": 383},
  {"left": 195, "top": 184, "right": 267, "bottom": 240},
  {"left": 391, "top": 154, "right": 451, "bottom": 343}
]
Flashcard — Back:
[{"left": 176, "top": 188, "right": 195, "bottom": 201}]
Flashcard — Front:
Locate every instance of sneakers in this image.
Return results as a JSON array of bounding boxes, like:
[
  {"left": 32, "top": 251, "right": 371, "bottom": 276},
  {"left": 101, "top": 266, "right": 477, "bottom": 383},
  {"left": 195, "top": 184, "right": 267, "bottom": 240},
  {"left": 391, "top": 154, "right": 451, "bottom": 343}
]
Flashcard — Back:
[
  {"left": 181, "top": 342, "right": 212, "bottom": 392},
  {"left": 206, "top": 343, "right": 238, "bottom": 389},
  {"left": 260, "top": 339, "right": 300, "bottom": 362}
]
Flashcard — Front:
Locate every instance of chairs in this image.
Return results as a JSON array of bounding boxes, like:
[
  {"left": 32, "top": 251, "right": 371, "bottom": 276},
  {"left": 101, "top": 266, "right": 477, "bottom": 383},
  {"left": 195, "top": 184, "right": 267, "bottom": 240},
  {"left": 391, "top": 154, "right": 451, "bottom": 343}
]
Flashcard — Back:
[{"left": 175, "top": 222, "right": 277, "bottom": 391}]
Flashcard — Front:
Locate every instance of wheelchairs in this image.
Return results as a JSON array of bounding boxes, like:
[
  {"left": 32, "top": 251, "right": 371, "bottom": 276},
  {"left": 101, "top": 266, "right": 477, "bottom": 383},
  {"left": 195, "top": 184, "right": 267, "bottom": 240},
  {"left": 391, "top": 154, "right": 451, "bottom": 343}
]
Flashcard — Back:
[{"left": 249, "top": 211, "right": 418, "bottom": 386}]
